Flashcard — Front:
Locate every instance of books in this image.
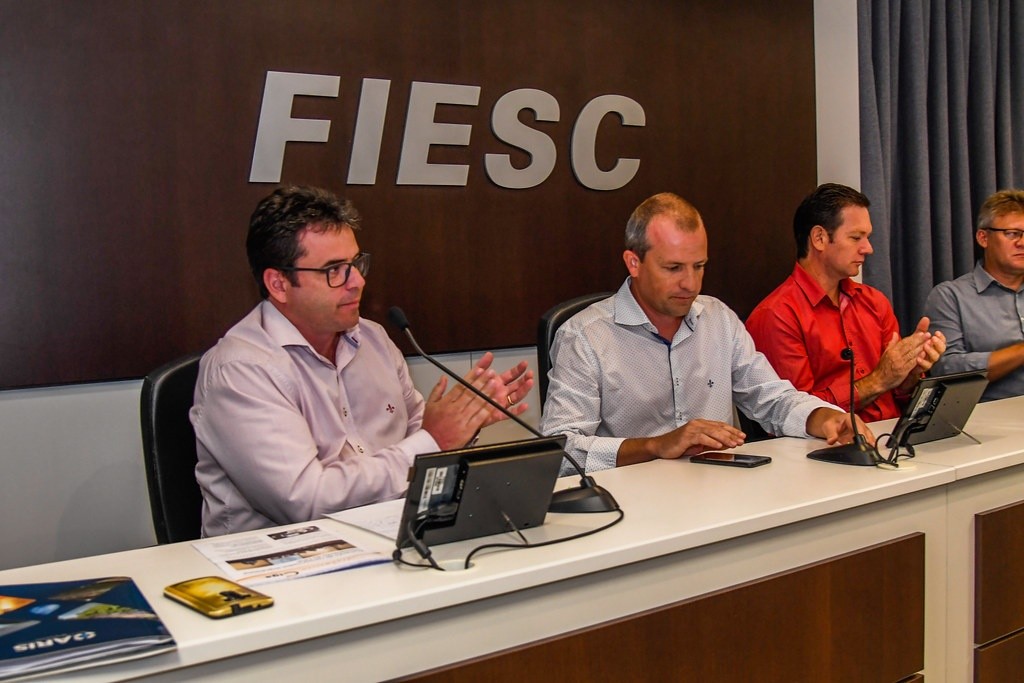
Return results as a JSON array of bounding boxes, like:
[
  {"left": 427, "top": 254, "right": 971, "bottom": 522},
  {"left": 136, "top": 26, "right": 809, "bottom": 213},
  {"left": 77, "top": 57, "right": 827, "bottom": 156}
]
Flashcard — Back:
[{"left": 0, "top": 575, "right": 179, "bottom": 683}]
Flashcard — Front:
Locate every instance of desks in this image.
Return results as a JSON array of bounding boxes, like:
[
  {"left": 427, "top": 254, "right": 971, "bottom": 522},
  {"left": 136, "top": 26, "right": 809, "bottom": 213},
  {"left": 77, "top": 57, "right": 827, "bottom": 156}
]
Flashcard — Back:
[
  {"left": 0, "top": 436, "right": 955, "bottom": 683},
  {"left": 864, "top": 396, "right": 1024, "bottom": 683}
]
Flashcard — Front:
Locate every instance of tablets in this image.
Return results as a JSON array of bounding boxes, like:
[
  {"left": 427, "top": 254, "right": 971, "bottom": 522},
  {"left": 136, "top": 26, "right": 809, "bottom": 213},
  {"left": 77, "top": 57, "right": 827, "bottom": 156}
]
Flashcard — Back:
[
  {"left": 886, "top": 368, "right": 989, "bottom": 448},
  {"left": 397, "top": 435, "right": 568, "bottom": 549}
]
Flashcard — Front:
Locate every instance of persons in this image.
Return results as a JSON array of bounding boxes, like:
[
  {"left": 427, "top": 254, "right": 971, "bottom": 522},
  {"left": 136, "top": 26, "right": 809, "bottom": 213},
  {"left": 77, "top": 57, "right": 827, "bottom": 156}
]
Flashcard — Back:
[
  {"left": 538, "top": 191, "right": 877, "bottom": 477},
  {"left": 918, "top": 184, "right": 1024, "bottom": 404},
  {"left": 188, "top": 179, "right": 536, "bottom": 545},
  {"left": 743, "top": 180, "right": 949, "bottom": 428}
]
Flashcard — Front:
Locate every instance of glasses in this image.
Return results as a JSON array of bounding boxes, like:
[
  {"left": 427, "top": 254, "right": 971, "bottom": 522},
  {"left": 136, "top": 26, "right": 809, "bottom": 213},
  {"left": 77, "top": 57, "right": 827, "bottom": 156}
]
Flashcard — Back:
[
  {"left": 267, "top": 252, "right": 371, "bottom": 289},
  {"left": 980, "top": 227, "right": 1024, "bottom": 239}
]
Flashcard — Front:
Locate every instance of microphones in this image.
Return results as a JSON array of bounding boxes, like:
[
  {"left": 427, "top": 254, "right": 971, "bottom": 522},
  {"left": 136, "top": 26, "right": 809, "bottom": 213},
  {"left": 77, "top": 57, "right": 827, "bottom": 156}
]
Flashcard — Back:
[
  {"left": 385, "top": 305, "right": 620, "bottom": 514},
  {"left": 807, "top": 348, "right": 882, "bottom": 466}
]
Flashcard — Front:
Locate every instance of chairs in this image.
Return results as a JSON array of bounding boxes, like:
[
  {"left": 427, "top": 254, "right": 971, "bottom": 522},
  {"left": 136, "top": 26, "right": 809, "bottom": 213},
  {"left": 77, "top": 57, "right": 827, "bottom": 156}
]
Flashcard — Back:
[
  {"left": 140, "top": 352, "right": 203, "bottom": 545},
  {"left": 536, "top": 292, "right": 615, "bottom": 417}
]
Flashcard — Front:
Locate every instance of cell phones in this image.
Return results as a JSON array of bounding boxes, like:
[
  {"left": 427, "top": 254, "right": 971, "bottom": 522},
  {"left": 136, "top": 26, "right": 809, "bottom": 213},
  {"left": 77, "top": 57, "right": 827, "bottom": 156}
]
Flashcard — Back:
[
  {"left": 163, "top": 575, "right": 274, "bottom": 618},
  {"left": 690, "top": 452, "right": 772, "bottom": 468}
]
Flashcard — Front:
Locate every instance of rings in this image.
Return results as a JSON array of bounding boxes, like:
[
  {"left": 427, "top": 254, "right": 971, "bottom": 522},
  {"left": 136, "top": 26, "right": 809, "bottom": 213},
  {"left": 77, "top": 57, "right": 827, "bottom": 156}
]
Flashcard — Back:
[{"left": 508, "top": 395, "right": 515, "bottom": 405}]
